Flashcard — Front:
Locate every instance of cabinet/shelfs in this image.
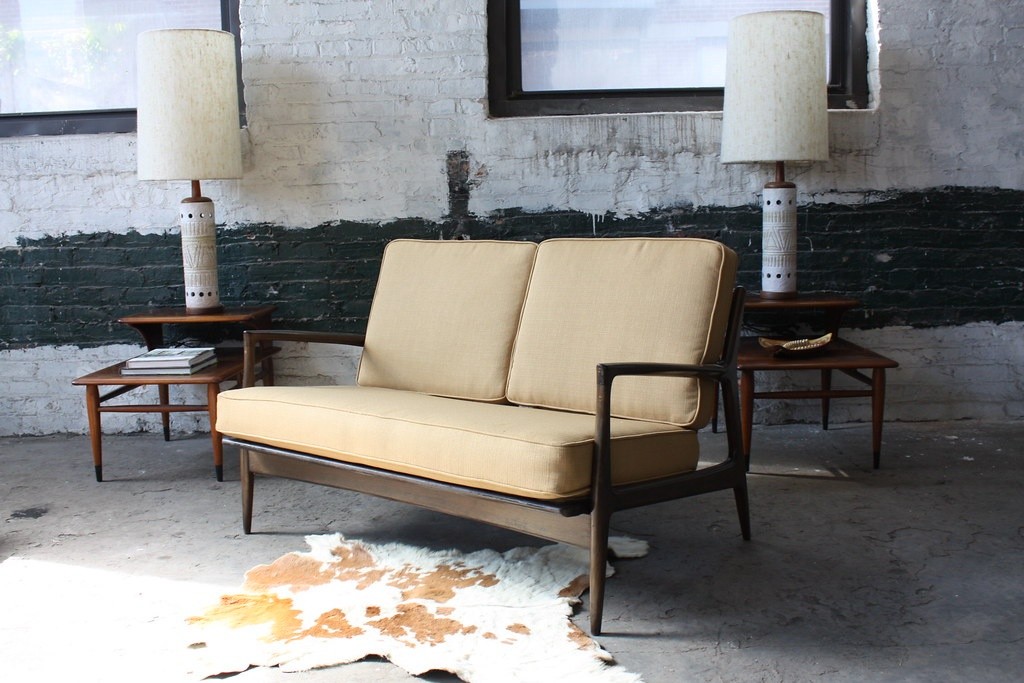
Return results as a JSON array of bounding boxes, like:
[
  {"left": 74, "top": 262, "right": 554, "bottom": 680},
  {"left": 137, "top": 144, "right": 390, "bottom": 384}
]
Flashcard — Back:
[
  {"left": 738, "top": 294, "right": 899, "bottom": 470},
  {"left": 71, "top": 307, "right": 281, "bottom": 483}
]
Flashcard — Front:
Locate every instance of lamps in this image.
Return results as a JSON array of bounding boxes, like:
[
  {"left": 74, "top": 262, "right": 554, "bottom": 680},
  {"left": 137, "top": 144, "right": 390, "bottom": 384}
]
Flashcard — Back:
[
  {"left": 719, "top": 10, "right": 831, "bottom": 300},
  {"left": 135, "top": 28, "right": 244, "bottom": 316}
]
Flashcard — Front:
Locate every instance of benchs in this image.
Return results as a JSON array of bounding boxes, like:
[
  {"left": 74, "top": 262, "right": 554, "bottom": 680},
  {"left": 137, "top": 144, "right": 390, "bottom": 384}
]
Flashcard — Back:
[{"left": 214, "top": 237, "right": 754, "bottom": 635}]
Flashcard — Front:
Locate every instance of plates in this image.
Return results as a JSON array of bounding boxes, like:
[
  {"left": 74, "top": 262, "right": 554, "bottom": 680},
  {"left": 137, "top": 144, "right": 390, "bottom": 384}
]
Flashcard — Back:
[{"left": 758, "top": 332, "right": 833, "bottom": 356}]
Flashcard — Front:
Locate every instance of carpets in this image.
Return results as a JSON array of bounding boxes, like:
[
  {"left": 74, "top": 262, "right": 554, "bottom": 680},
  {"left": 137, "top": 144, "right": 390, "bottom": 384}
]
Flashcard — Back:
[{"left": 181, "top": 532, "right": 649, "bottom": 683}]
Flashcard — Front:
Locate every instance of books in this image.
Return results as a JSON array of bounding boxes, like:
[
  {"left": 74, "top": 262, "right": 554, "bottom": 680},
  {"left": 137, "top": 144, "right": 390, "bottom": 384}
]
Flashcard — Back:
[{"left": 119, "top": 347, "right": 217, "bottom": 375}]
